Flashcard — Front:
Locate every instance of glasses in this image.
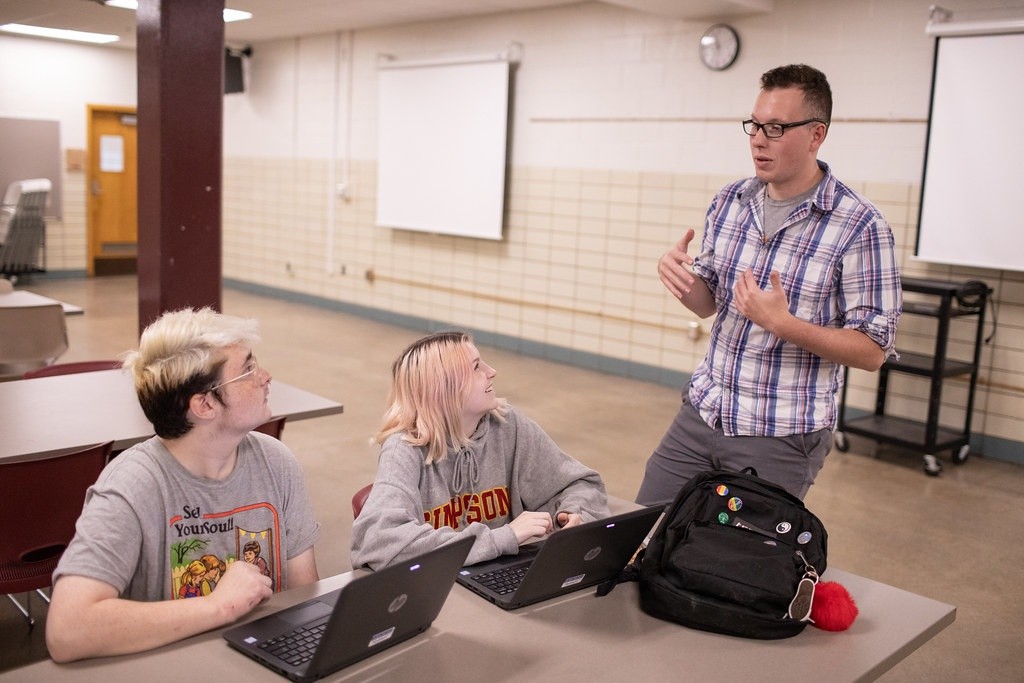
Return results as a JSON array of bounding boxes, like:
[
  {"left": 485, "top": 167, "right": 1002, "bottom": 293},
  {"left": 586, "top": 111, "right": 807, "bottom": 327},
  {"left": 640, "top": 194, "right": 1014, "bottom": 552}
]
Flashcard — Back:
[
  {"left": 201, "top": 354, "right": 261, "bottom": 396},
  {"left": 742, "top": 119, "right": 829, "bottom": 139}
]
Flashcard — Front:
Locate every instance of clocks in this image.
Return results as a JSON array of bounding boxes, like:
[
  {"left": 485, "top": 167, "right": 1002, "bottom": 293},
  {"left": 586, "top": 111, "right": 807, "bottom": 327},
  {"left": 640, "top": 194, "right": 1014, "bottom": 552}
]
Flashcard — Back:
[{"left": 697, "top": 21, "right": 740, "bottom": 71}]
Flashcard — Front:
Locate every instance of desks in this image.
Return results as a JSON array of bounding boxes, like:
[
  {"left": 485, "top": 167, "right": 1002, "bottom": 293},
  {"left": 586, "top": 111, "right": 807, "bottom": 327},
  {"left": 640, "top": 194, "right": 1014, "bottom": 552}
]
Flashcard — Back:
[
  {"left": 0, "top": 370, "right": 343, "bottom": 462},
  {"left": 0, "top": 492, "right": 956, "bottom": 683},
  {"left": 0, "top": 290, "right": 86, "bottom": 315}
]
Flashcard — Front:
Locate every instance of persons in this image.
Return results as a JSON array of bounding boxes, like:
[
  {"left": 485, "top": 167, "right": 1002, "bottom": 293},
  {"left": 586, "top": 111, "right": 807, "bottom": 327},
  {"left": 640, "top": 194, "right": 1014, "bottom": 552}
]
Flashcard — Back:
[
  {"left": 635, "top": 63, "right": 901, "bottom": 508},
  {"left": 45, "top": 305, "right": 322, "bottom": 663},
  {"left": 349, "top": 332, "right": 611, "bottom": 574}
]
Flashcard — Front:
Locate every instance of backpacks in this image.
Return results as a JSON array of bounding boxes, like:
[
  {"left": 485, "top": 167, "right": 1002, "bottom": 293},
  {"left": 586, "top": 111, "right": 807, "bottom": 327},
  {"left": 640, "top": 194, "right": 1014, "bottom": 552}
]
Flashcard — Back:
[{"left": 626, "top": 467, "right": 861, "bottom": 641}]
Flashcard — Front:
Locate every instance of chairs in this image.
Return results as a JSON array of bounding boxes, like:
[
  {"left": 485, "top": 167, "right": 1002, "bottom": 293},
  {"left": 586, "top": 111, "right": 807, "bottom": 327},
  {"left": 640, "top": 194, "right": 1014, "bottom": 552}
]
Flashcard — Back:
[
  {"left": 0, "top": 278, "right": 69, "bottom": 382},
  {"left": 1, "top": 177, "right": 51, "bottom": 273},
  {"left": 0, "top": 360, "right": 286, "bottom": 628}
]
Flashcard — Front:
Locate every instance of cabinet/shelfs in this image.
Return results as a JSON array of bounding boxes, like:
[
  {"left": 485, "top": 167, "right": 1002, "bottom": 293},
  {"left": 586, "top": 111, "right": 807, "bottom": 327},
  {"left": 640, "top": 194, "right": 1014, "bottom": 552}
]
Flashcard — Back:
[{"left": 834, "top": 275, "right": 988, "bottom": 475}]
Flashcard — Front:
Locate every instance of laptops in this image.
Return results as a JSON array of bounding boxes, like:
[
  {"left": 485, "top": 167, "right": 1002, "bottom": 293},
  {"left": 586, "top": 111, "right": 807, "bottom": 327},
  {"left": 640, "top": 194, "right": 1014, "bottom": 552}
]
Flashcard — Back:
[
  {"left": 222, "top": 534, "right": 479, "bottom": 683},
  {"left": 458, "top": 503, "right": 668, "bottom": 609}
]
